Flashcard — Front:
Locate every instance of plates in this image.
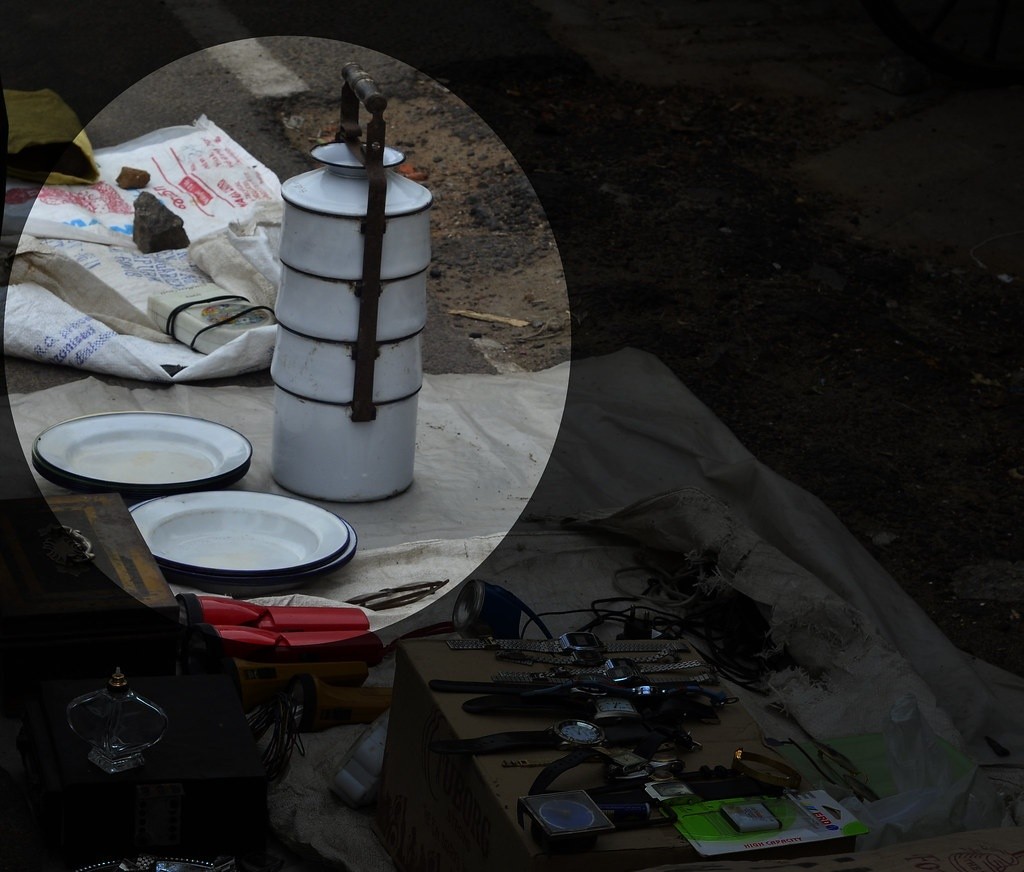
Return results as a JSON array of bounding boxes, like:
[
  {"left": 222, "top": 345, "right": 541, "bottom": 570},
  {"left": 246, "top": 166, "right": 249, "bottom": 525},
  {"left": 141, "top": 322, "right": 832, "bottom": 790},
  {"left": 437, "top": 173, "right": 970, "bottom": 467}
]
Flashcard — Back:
[
  {"left": 32, "top": 411, "right": 252, "bottom": 502},
  {"left": 129, "top": 490, "right": 357, "bottom": 586}
]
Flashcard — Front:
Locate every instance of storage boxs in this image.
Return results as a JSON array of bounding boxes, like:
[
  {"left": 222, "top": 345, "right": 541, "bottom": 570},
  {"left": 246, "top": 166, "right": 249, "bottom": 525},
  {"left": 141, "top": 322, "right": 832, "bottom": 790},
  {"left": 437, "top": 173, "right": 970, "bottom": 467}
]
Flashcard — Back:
[
  {"left": 372, "top": 636, "right": 857, "bottom": 872},
  {"left": 635, "top": 821, "right": 1024, "bottom": 872}
]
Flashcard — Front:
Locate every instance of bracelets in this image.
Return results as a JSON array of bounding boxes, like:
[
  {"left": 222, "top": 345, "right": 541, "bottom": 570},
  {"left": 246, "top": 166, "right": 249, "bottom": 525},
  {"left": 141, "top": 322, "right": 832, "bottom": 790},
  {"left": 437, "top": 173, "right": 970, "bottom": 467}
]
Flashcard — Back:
[{"left": 732, "top": 747, "right": 802, "bottom": 788}]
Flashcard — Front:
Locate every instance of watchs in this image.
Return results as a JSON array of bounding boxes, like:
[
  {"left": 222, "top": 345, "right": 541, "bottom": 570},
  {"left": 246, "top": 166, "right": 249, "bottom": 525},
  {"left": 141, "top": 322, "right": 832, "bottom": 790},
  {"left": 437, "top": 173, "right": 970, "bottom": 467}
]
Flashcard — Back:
[{"left": 430, "top": 632, "right": 775, "bottom": 806}]
